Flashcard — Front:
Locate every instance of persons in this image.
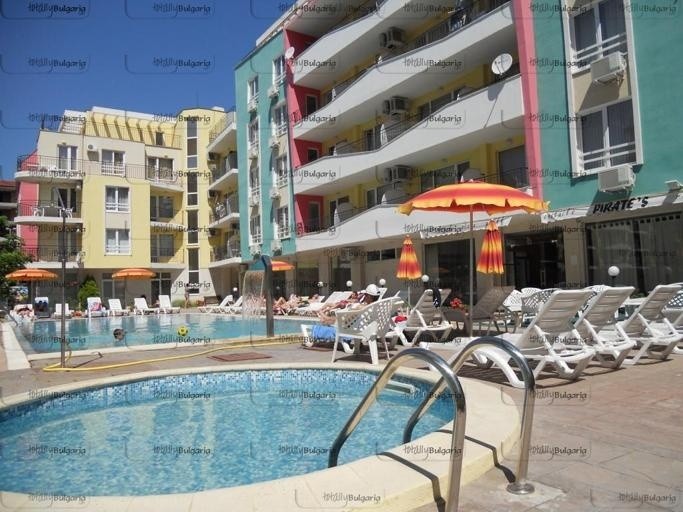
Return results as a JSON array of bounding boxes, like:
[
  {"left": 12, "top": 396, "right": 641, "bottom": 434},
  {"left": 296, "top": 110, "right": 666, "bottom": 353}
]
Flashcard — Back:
[
  {"left": 35, "top": 300, "right": 46, "bottom": 312},
  {"left": 183, "top": 283, "right": 192, "bottom": 309},
  {"left": 272, "top": 283, "right": 381, "bottom": 327},
  {"left": 113, "top": 329, "right": 126, "bottom": 344},
  {"left": 92, "top": 301, "right": 103, "bottom": 311}
]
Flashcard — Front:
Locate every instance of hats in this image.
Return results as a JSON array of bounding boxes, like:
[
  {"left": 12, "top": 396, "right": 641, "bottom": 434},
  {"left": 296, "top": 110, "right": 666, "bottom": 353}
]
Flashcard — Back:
[{"left": 361, "top": 283, "right": 381, "bottom": 297}]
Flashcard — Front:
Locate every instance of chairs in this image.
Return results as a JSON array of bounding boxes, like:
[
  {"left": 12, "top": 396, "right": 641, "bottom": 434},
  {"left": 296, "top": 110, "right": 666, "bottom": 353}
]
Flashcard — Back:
[{"left": 30, "top": 205, "right": 74, "bottom": 218}]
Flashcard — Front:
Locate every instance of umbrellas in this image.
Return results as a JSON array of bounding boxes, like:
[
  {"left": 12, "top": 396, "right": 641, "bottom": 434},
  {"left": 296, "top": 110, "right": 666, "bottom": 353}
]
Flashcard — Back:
[
  {"left": 272, "top": 259, "right": 295, "bottom": 272},
  {"left": 394, "top": 175, "right": 550, "bottom": 339},
  {"left": 111, "top": 267, "right": 156, "bottom": 307},
  {"left": 5, "top": 268, "right": 58, "bottom": 304}
]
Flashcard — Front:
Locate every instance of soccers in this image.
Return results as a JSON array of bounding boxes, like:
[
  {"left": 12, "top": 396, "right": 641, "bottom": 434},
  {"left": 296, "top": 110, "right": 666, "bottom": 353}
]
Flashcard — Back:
[{"left": 178, "top": 327, "right": 189, "bottom": 337}]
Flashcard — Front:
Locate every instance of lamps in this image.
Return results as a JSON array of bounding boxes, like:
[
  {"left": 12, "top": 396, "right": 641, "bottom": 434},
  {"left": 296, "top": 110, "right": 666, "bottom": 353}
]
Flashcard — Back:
[{"left": 665, "top": 180, "right": 682, "bottom": 190}]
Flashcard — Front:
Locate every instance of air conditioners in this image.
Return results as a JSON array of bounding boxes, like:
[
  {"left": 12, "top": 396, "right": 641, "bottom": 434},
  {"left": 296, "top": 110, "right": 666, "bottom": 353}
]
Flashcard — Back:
[
  {"left": 87, "top": 144, "right": 99, "bottom": 152},
  {"left": 378, "top": 26, "right": 414, "bottom": 184},
  {"left": 598, "top": 165, "right": 636, "bottom": 195},
  {"left": 246, "top": 86, "right": 282, "bottom": 256},
  {"left": 590, "top": 51, "right": 627, "bottom": 87},
  {"left": 207, "top": 151, "right": 218, "bottom": 239}
]
